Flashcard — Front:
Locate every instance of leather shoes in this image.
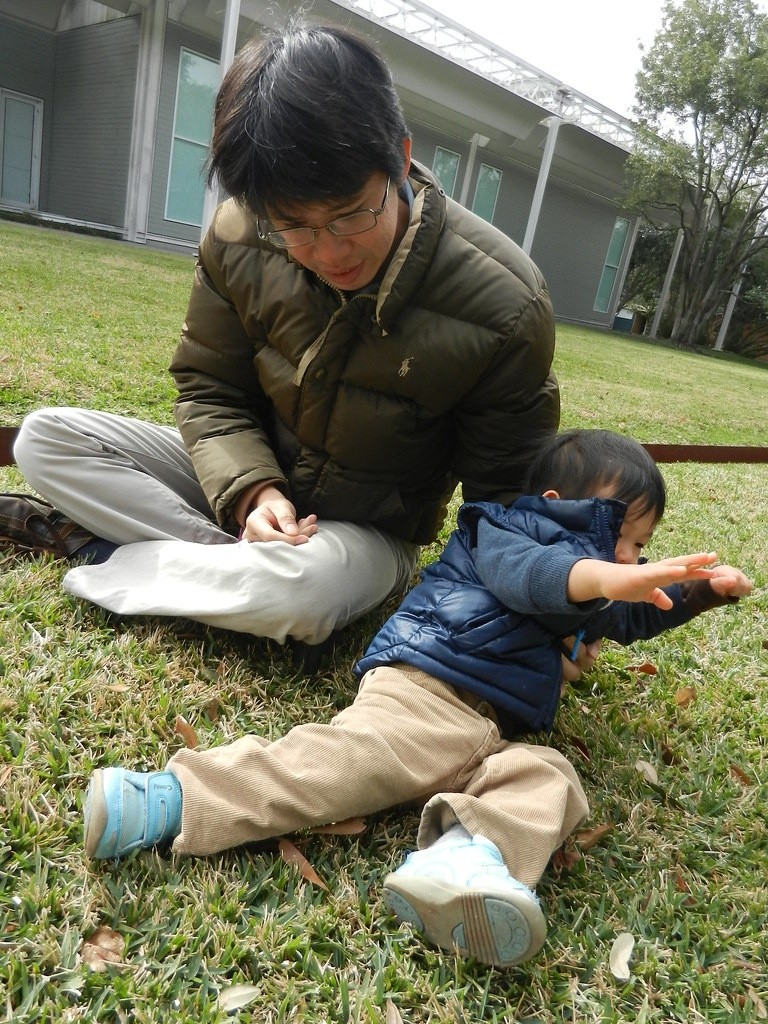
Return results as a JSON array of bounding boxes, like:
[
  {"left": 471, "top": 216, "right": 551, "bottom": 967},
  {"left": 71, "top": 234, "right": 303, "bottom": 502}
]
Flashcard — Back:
[{"left": 0, "top": 492, "right": 94, "bottom": 559}]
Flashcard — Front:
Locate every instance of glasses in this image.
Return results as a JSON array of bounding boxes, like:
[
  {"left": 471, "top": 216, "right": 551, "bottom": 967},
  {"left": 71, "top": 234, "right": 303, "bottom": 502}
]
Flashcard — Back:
[{"left": 256, "top": 175, "right": 391, "bottom": 249}]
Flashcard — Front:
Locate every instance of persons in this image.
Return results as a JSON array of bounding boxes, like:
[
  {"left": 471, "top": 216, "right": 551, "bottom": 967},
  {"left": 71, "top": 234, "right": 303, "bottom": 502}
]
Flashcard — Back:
[
  {"left": 83, "top": 433, "right": 758, "bottom": 968},
  {"left": 0, "top": 25, "right": 605, "bottom": 698}
]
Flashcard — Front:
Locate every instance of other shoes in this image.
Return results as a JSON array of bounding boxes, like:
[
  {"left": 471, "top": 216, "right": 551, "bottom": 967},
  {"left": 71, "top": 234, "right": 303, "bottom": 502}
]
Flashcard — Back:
[
  {"left": 381, "top": 836, "right": 545, "bottom": 967},
  {"left": 82, "top": 768, "right": 181, "bottom": 860}
]
